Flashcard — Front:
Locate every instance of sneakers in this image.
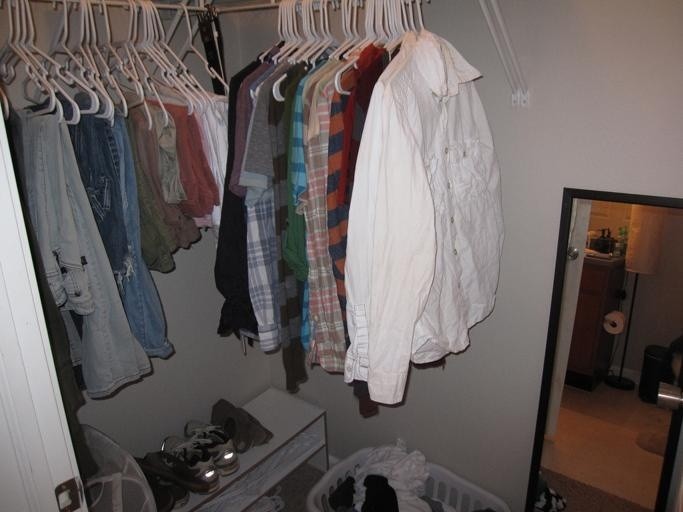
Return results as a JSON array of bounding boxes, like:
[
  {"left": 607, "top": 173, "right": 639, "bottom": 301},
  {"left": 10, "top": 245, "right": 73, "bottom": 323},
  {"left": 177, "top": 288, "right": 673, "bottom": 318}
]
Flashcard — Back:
[
  {"left": 211, "top": 399, "right": 252, "bottom": 454},
  {"left": 185, "top": 419, "right": 240, "bottom": 476},
  {"left": 236, "top": 408, "right": 273, "bottom": 446},
  {"left": 131, "top": 457, "right": 175, "bottom": 512},
  {"left": 161, "top": 435, "right": 220, "bottom": 495},
  {"left": 144, "top": 449, "right": 210, "bottom": 494}
]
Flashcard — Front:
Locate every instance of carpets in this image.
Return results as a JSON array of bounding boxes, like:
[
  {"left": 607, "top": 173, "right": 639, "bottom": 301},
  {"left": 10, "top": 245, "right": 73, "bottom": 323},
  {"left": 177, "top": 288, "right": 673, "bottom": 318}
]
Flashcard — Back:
[{"left": 537, "top": 466, "right": 651, "bottom": 512}]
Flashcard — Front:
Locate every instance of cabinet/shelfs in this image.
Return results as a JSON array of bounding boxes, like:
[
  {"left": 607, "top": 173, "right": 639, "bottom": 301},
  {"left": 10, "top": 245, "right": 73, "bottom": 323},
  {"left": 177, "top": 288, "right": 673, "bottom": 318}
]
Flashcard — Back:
[
  {"left": 566, "top": 254, "right": 627, "bottom": 393},
  {"left": 160, "top": 389, "right": 329, "bottom": 512}
]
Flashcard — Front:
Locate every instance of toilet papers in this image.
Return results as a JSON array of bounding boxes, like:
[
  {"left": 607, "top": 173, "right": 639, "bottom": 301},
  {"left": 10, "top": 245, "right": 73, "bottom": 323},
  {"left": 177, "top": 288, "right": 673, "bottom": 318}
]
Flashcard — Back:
[{"left": 604, "top": 312, "right": 625, "bottom": 335}]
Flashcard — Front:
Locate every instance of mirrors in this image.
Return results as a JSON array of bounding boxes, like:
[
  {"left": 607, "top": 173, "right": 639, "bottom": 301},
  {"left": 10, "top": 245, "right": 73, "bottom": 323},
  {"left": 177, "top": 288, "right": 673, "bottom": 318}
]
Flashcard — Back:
[{"left": 525, "top": 187, "right": 683, "bottom": 512}]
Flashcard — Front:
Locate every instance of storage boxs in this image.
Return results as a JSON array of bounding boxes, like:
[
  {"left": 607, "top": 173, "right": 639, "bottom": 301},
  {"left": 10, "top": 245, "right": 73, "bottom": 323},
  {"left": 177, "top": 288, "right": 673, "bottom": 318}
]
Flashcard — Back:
[{"left": 308, "top": 447, "right": 509, "bottom": 512}]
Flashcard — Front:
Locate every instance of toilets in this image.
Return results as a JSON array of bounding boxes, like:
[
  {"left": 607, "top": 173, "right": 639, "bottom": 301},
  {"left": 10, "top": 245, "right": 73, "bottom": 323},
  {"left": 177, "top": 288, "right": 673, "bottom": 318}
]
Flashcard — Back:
[{"left": 672, "top": 353, "right": 682, "bottom": 386}]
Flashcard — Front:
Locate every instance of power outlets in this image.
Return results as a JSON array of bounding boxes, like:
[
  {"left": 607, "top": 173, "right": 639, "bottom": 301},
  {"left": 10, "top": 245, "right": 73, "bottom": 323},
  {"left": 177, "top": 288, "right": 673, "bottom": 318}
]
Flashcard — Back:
[{"left": 621, "top": 286, "right": 627, "bottom": 304}]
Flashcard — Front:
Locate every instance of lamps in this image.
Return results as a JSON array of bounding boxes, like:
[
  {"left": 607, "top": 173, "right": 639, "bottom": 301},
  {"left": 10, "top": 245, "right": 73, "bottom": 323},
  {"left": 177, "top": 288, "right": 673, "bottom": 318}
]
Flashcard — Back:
[{"left": 606, "top": 205, "right": 663, "bottom": 391}]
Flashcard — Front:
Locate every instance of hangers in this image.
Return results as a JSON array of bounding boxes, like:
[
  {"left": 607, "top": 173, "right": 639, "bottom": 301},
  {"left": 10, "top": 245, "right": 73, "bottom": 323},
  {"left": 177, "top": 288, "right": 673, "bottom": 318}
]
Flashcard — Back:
[
  {"left": 0, "top": 0, "right": 230, "bottom": 128},
  {"left": 232, "top": 0, "right": 460, "bottom": 100}
]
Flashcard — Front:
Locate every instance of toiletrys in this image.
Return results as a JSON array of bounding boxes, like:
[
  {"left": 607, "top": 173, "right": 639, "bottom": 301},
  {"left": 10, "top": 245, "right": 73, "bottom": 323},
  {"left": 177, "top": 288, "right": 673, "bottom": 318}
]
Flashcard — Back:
[
  {"left": 605, "top": 227, "right": 613, "bottom": 252},
  {"left": 598, "top": 228, "right": 609, "bottom": 253}
]
